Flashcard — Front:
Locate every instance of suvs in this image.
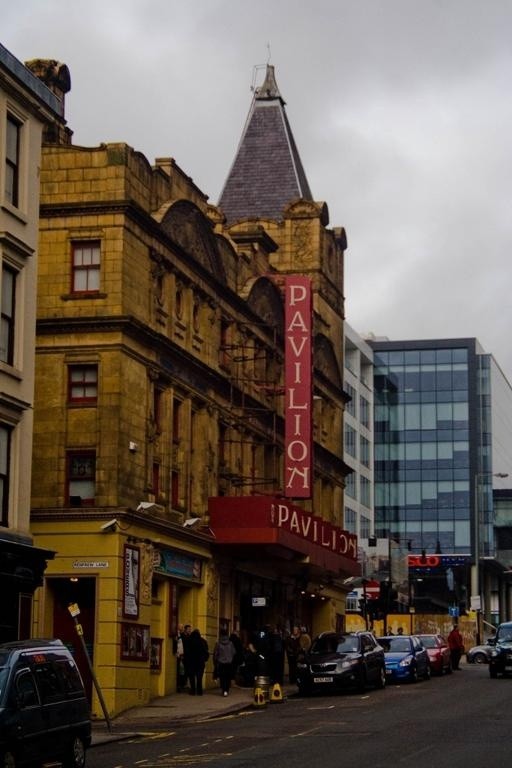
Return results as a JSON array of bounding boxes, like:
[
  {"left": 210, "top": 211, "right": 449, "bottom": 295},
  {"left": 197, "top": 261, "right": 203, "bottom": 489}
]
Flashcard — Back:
[{"left": 293, "top": 629, "right": 387, "bottom": 693}]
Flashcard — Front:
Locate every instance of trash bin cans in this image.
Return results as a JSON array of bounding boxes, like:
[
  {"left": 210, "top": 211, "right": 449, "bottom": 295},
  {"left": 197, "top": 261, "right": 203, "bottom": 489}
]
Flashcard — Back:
[{"left": 253, "top": 676, "right": 270, "bottom": 701}]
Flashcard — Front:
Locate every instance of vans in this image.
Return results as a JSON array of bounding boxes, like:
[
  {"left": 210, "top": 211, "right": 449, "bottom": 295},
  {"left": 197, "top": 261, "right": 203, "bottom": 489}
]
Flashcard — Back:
[{"left": 0, "top": 636, "right": 93, "bottom": 768}]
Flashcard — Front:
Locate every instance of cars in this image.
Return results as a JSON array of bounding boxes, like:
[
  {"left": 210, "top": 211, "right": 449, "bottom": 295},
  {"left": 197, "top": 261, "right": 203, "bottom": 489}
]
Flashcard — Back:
[
  {"left": 412, "top": 634, "right": 455, "bottom": 677},
  {"left": 466, "top": 634, "right": 497, "bottom": 664},
  {"left": 375, "top": 635, "right": 433, "bottom": 685},
  {"left": 487, "top": 621, "right": 512, "bottom": 679}
]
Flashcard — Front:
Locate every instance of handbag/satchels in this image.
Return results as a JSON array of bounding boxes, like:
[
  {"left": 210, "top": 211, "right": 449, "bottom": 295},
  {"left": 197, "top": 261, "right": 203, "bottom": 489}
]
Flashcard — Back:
[
  {"left": 213, "top": 664, "right": 219, "bottom": 679},
  {"left": 179, "top": 660, "right": 185, "bottom": 675}
]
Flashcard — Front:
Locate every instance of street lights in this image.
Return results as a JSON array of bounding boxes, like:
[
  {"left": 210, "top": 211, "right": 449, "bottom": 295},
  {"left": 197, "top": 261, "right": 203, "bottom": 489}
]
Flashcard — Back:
[{"left": 473, "top": 470, "right": 510, "bottom": 644}]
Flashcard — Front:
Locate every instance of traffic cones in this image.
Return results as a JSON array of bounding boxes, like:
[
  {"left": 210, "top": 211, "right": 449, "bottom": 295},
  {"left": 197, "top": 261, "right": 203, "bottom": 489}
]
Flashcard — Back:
[
  {"left": 269, "top": 679, "right": 288, "bottom": 703},
  {"left": 250, "top": 686, "right": 267, "bottom": 708}
]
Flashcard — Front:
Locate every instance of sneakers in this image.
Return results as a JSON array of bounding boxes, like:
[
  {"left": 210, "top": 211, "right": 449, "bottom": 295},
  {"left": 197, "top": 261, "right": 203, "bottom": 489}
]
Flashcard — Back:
[
  {"left": 178, "top": 687, "right": 203, "bottom": 696},
  {"left": 224, "top": 690, "right": 228, "bottom": 696},
  {"left": 453, "top": 667, "right": 462, "bottom": 671}
]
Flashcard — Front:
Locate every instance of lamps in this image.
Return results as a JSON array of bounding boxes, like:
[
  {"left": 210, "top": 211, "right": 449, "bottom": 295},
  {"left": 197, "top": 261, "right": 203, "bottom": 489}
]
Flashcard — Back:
[
  {"left": 181, "top": 517, "right": 201, "bottom": 528},
  {"left": 135, "top": 500, "right": 154, "bottom": 513}
]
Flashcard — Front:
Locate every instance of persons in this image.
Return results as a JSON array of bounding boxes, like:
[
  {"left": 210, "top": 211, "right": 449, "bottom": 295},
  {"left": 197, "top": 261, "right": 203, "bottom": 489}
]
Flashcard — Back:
[
  {"left": 385, "top": 625, "right": 395, "bottom": 635},
  {"left": 175, "top": 621, "right": 313, "bottom": 697},
  {"left": 456, "top": 628, "right": 465, "bottom": 670},
  {"left": 396, "top": 626, "right": 404, "bottom": 635},
  {"left": 370, "top": 629, "right": 377, "bottom": 637},
  {"left": 368, "top": 625, "right": 375, "bottom": 632},
  {"left": 448, "top": 623, "right": 462, "bottom": 670}
]
